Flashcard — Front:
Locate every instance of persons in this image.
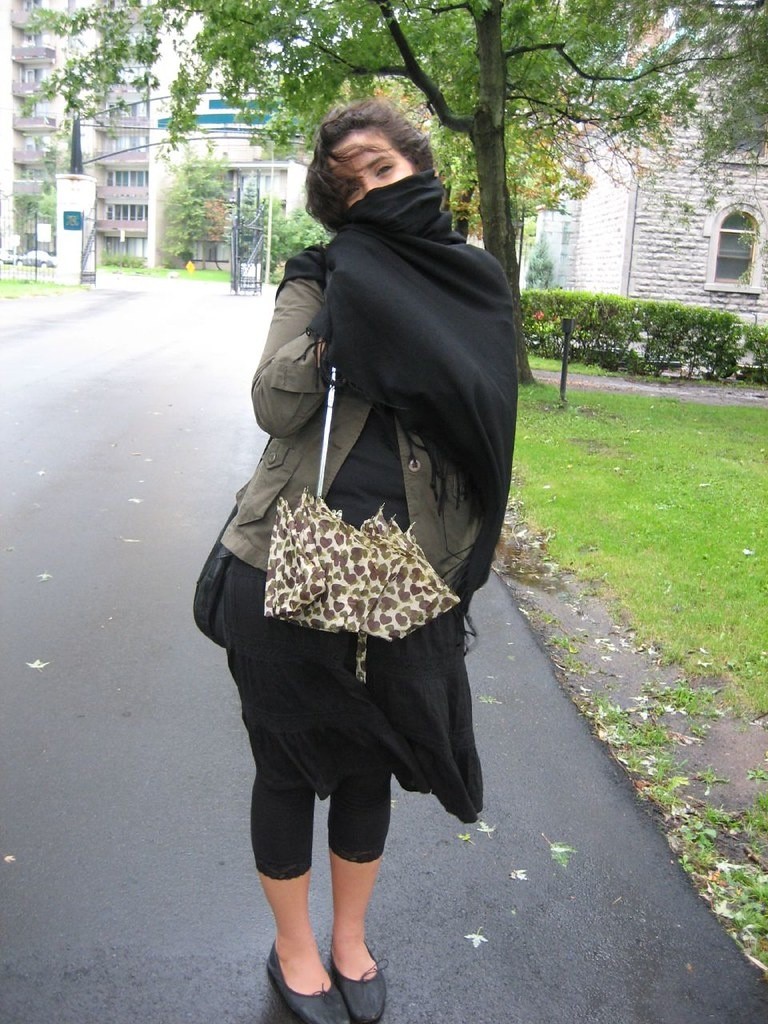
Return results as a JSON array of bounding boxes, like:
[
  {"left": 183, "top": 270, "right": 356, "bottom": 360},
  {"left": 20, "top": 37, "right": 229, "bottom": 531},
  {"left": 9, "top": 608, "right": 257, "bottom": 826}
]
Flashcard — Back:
[{"left": 220, "top": 100, "right": 518, "bottom": 1023}]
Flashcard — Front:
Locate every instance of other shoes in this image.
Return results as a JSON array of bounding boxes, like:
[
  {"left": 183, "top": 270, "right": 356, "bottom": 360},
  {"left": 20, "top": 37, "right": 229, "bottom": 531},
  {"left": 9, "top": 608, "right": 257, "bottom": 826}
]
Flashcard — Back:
[
  {"left": 330, "top": 937, "right": 389, "bottom": 1024},
  {"left": 268, "top": 939, "right": 350, "bottom": 1024}
]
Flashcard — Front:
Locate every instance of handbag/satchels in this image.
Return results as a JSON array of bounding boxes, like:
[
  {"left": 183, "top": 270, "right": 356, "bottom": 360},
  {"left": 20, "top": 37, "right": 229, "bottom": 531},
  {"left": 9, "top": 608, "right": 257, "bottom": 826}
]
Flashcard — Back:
[{"left": 193, "top": 503, "right": 237, "bottom": 649}]
